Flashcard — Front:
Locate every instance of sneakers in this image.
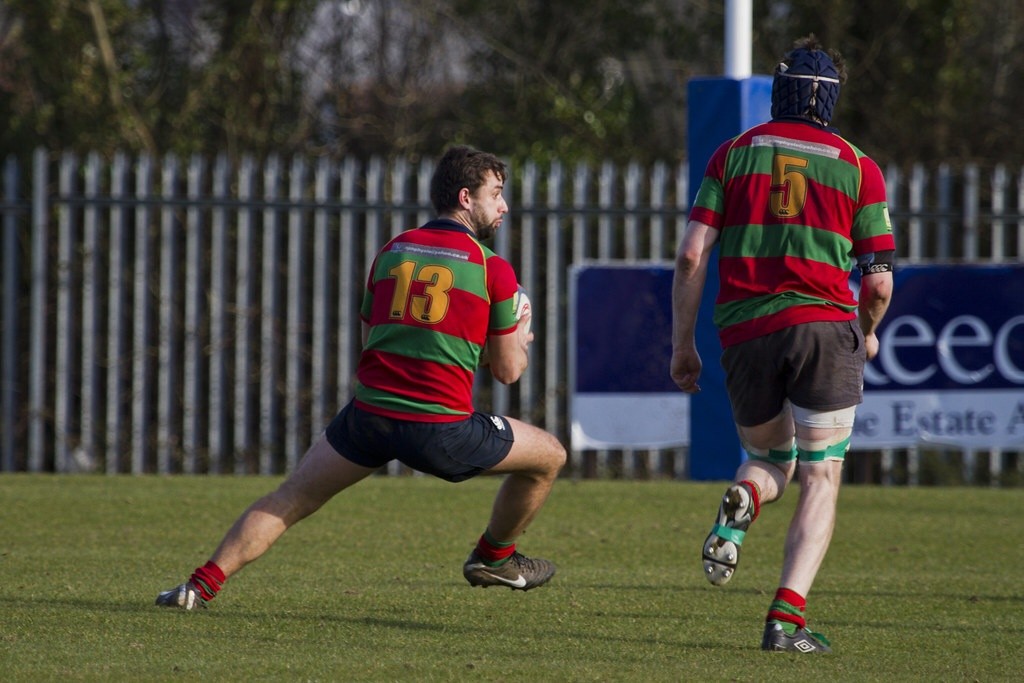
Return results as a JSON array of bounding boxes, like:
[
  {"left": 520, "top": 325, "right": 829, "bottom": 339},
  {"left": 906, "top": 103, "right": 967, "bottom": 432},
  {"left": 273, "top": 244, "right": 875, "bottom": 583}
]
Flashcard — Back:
[
  {"left": 155, "top": 582, "right": 208, "bottom": 612},
  {"left": 462, "top": 548, "right": 557, "bottom": 592},
  {"left": 701, "top": 482, "right": 756, "bottom": 586},
  {"left": 762, "top": 622, "right": 832, "bottom": 654}
]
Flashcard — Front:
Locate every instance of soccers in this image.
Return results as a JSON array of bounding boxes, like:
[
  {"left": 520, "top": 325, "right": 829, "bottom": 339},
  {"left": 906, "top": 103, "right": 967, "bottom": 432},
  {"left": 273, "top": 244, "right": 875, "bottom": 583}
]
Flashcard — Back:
[{"left": 481, "top": 285, "right": 532, "bottom": 360}]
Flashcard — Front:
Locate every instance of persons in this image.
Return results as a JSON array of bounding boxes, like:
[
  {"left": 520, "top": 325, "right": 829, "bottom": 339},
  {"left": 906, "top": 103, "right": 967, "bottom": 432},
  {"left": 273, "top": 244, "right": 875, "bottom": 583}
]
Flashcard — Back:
[
  {"left": 668, "top": 33, "right": 897, "bottom": 655},
  {"left": 154, "top": 144, "right": 567, "bottom": 609}
]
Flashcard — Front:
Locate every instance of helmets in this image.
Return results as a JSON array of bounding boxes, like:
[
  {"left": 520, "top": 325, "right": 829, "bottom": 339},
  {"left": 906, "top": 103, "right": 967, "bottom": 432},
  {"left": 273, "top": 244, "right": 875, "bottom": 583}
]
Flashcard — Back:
[{"left": 770, "top": 49, "right": 841, "bottom": 126}]
